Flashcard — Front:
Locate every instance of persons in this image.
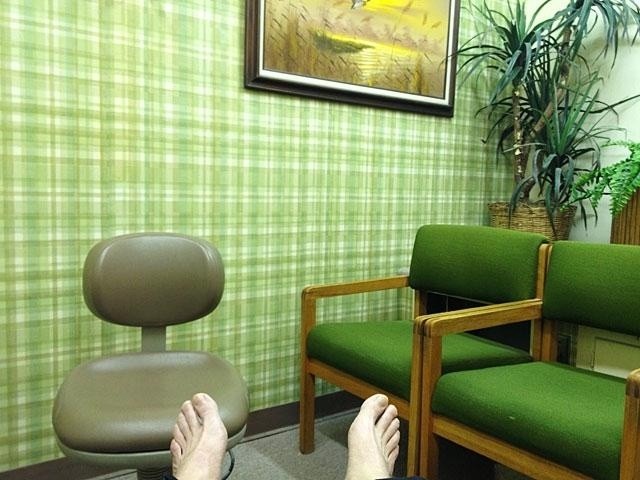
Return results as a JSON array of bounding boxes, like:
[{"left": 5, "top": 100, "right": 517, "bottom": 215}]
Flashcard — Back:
[{"left": 169, "top": 392, "right": 401, "bottom": 480}]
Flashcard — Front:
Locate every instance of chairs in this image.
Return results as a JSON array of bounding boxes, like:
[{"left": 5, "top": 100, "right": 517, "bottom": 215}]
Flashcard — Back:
[
  {"left": 417, "top": 240, "right": 639, "bottom": 479},
  {"left": 57, "top": 226, "right": 249, "bottom": 479},
  {"left": 297, "top": 220, "right": 550, "bottom": 479}
]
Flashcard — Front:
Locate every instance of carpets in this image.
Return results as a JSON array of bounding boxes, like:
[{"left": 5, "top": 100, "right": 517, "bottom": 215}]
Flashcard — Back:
[{"left": 82, "top": 405, "right": 533, "bottom": 479}]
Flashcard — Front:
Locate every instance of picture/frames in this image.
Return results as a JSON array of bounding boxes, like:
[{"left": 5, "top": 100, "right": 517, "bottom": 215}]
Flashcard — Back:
[{"left": 241, "top": 0, "right": 460, "bottom": 117}]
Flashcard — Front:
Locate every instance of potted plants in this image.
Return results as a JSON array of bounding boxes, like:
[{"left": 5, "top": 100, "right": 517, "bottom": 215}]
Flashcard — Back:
[{"left": 445, "top": 1, "right": 638, "bottom": 239}]
